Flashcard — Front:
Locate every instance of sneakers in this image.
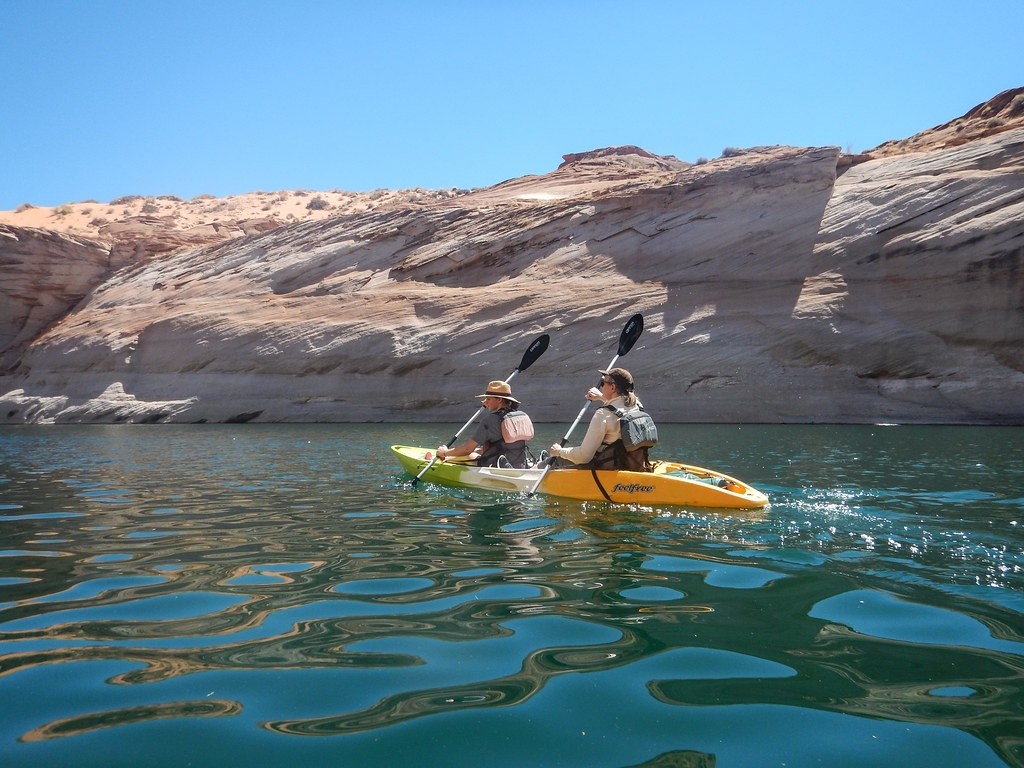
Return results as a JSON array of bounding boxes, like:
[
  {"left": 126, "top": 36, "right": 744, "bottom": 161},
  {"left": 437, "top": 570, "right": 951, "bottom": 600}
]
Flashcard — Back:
[
  {"left": 539, "top": 450, "right": 548, "bottom": 460},
  {"left": 497, "top": 455, "right": 514, "bottom": 469}
]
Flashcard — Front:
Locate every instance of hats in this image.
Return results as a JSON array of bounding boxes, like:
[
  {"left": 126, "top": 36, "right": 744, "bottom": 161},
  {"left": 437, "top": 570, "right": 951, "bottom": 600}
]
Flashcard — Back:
[
  {"left": 474, "top": 380, "right": 521, "bottom": 405},
  {"left": 598, "top": 368, "right": 634, "bottom": 392}
]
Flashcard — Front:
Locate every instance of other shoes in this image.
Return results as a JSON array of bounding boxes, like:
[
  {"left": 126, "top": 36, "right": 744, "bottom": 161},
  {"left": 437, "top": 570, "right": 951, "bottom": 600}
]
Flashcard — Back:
[{"left": 425, "top": 452, "right": 433, "bottom": 461}]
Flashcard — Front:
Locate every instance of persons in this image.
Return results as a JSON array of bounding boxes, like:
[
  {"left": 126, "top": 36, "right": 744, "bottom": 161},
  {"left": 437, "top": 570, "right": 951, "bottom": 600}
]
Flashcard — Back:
[
  {"left": 530, "top": 368, "right": 647, "bottom": 472},
  {"left": 436, "top": 380, "right": 527, "bottom": 469}
]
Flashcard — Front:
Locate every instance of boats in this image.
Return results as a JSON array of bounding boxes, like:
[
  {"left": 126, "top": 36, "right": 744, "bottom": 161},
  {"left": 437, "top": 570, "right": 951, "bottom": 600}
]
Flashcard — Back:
[{"left": 389, "top": 442, "right": 769, "bottom": 509}]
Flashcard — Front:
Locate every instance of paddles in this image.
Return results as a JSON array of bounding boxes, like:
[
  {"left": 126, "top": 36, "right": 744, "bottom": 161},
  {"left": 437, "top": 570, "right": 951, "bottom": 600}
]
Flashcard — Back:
[
  {"left": 410, "top": 331, "right": 551, "bottom": 487},
  {"left": 526, "top": 312, "right": 645, "bottom": 500}
]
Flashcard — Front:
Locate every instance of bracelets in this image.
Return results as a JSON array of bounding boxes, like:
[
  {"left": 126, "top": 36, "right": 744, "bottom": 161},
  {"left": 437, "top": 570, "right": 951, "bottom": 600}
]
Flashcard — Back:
[{"left": 443, "top": 449, "right": 448, "bottom": 457}]
